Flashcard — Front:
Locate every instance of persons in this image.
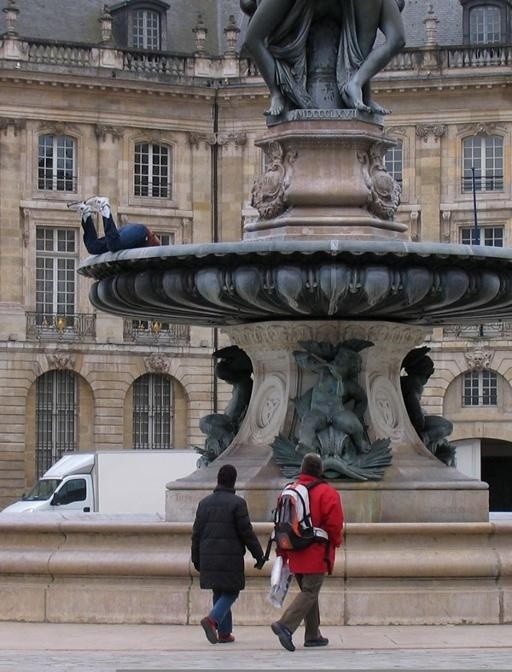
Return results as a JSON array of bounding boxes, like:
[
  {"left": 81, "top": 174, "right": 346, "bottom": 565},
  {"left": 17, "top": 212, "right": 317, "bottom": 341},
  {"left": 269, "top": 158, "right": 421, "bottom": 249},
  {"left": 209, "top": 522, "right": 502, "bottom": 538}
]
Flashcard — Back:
[
  {"left": 66, "top": 197, "right": 163, "bottom": 255},
  {"left": 271, "top": 452, "right": 342, "bottom": 652},
  {"left": 190, "top": 463, "right": 265, "bottom": 644},
  {"left": 292, "top": 348, "right": 371, "bottom": 455},
  {"left": 344, "top": 1, "right": 406, "bottom": 115},
  {"left": 242, "top": 1, "right": 301, "bottom": 117}
]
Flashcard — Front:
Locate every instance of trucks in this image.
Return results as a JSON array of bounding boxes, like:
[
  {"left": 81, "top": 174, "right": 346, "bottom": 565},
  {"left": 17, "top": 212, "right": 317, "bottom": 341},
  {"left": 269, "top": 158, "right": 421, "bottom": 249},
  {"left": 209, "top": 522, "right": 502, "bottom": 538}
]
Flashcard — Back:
[{"left": 0, "top": 448, "right": 204, "bottom": 529}]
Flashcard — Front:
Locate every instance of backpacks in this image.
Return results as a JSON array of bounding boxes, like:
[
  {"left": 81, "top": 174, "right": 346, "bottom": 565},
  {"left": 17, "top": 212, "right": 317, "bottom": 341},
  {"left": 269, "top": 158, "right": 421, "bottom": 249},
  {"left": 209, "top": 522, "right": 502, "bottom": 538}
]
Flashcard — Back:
[{"left": 274, "top": 479, "right": 330, "bottom": 550}]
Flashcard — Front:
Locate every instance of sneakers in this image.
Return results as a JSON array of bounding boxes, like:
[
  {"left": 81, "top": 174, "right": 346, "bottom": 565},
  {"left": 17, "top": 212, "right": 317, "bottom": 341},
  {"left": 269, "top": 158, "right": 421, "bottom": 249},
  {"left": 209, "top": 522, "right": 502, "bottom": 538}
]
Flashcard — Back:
[
  {"left": 85, "top": 196, "right": 110, "bottom": 209},
  {"left": 67, "top": 201, "right": 89, "bottom": 214},
  {"left": 219, "top": 632, "right": 235, "bottom": 642},
  {"left": 272, "top": 621, "right": 297, "bottom": 655},
  {"left": 200, "top": 617, "right": 219, "bottom": 643},
  {"left": 303, "top": 634, "right": 331, "bottom": 650}
]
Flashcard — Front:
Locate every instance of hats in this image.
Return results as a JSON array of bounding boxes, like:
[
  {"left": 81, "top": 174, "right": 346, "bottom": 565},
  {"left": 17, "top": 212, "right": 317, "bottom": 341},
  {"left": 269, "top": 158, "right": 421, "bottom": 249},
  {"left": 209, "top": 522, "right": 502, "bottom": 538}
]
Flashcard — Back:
[{"left": 302, "top": 453, "right": 322, "bottom": 473}]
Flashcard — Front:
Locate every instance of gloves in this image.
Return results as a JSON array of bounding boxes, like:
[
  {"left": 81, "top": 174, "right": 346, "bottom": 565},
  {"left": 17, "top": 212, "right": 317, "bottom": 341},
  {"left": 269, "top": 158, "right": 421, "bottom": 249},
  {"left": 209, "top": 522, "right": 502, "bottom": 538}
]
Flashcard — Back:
[
  {"left": 253, "top": 555, "right": 266, "bottom": 570},
  {"left": 193, "top": 561, "right": 201, "bottom": 572}
]
